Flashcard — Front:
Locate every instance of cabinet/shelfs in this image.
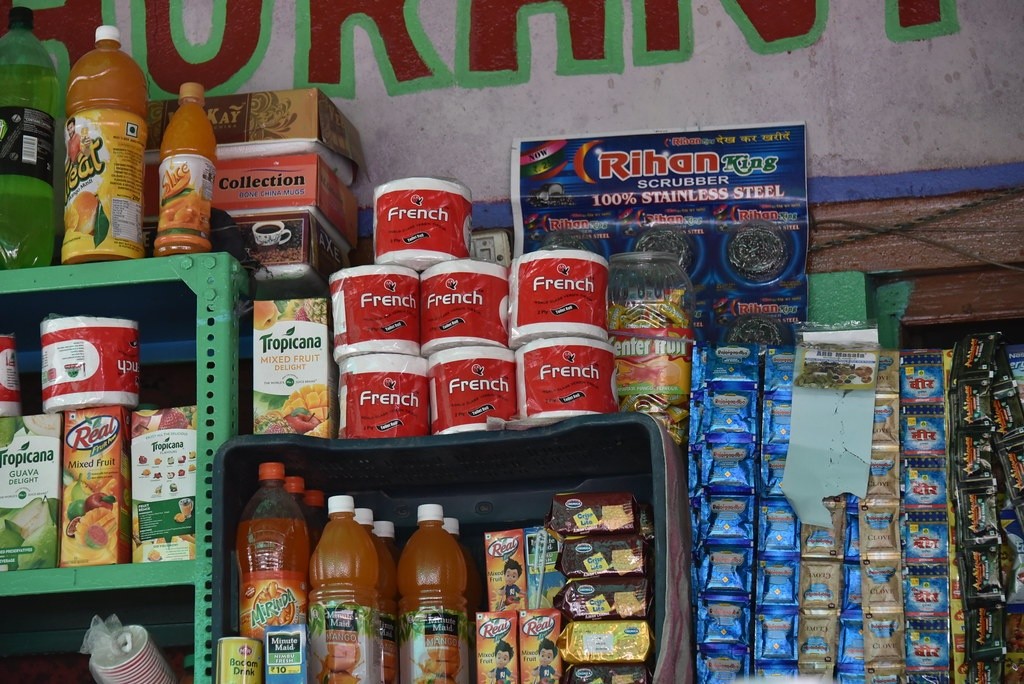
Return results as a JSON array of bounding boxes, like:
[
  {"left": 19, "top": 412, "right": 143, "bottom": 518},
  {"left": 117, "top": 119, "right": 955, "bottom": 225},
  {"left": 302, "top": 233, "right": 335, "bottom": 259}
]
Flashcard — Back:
[{"left": 0, "top": 251, "right": 237, "bottom": 683}]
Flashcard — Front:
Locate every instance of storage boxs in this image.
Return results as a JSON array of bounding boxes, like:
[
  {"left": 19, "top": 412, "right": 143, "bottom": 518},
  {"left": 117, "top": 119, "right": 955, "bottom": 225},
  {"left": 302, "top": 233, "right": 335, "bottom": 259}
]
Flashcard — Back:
[
  {"left": 134, "top": 88, "right": 368, "bottom": 293},
  {"left": 208, "top": 411, "right": 693, "bottom": 683}
]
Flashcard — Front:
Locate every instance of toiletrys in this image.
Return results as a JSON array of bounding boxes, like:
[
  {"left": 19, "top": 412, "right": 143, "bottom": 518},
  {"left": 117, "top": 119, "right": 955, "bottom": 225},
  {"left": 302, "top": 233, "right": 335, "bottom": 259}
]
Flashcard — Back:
[{"left": 685, "top": 330, "right": 1024, "bottom": 684}]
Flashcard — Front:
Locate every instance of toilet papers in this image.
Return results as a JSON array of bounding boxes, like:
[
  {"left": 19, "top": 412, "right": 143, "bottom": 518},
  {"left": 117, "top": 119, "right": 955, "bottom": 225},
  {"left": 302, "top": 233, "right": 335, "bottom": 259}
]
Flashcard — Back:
[
  {"left": 0, "top": 332, "right": 20, "bottom": 422},
  {"left": 325, "top": 170, "right": 616, "bottom": 435},
  {"left": 39, "top": 314, "right": 142, "bottom": 412}
]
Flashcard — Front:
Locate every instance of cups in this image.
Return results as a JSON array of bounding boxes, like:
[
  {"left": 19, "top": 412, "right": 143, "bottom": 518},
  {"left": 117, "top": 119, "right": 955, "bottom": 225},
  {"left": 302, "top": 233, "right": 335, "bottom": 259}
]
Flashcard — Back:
[{"left": 89, "top": 623, "right": 176, "bottom": 684}]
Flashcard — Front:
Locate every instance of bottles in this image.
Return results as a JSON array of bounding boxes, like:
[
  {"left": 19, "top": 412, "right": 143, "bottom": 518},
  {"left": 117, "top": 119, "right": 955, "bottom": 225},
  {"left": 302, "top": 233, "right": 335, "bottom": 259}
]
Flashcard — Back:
[
  {"left": 152, "top": 80, "right": 218, "bottom": 260},
  {"left": 61, "top": 25, "right": 149, "bottom": 265},
  {"left": 1, "top": 7, "right": 60, "bottom": 272},
  {"left": 237, "top": 462, "right": 472, "bottom": 684},
  {"left": 606, "top": 250, "right": 697, "bottom": 444}
]
